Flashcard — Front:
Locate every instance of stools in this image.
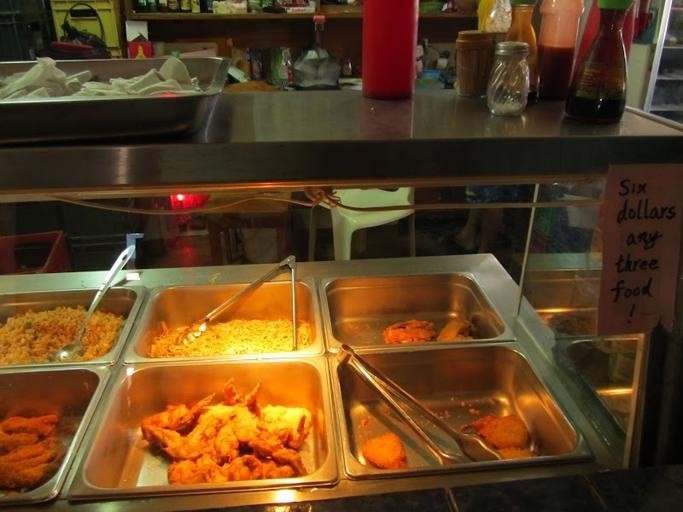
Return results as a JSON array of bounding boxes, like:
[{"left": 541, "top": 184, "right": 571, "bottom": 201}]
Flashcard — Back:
[
  {"left": 309, "top": 187, "right": 415, "bottom": 260},
  {"left": 199, "top": 193, "right": 291, "bottom": 265}
]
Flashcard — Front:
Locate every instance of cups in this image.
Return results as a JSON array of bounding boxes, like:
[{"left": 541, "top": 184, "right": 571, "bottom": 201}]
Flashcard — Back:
[
  {"left": 361, "top": 0, "right": 419, "bottom": 97},
  {"left": 358, "top": 100, "right": 413, "bottom": 141}
]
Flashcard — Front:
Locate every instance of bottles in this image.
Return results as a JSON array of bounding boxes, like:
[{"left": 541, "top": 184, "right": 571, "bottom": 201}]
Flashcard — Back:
[
  {"left": 129, "top": 0, "right": 212, "bottom": 14},
  {"left": 456, "top": 2, "right": 632, "bottom": 122},
  {"left": 292, "top": 16, "right": 337, "bottom": 92}
]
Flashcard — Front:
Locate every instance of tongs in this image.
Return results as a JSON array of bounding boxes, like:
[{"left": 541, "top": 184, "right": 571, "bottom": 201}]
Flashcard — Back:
[
  {"left": 337, "top": 340, "right": 499, "bottom": 466},
  {"left": 176, "top": 255, "right": 297, "bottom": 357}
]
